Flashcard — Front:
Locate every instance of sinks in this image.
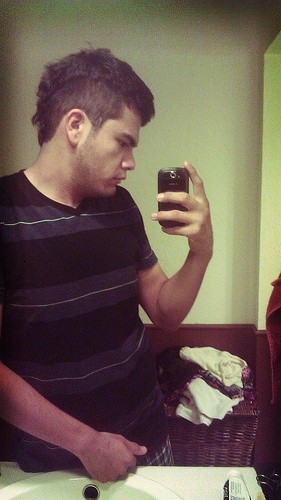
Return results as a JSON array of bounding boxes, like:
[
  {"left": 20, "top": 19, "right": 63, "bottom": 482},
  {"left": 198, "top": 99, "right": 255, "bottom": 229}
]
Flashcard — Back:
[{"left": 0, "top": 466, "right": 186, "bottom": 500}]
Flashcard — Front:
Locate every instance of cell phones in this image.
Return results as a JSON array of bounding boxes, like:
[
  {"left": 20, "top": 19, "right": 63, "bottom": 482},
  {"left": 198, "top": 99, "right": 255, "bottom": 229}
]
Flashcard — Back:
[{"left": 158, "top": 166, "right": 188, "bottom": 227}]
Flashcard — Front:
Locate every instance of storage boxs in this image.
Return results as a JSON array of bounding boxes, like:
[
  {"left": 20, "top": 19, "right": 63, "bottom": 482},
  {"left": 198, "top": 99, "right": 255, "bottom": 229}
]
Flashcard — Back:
[{"left": 162, "top": 403, "right": 259, "bottom": 468}]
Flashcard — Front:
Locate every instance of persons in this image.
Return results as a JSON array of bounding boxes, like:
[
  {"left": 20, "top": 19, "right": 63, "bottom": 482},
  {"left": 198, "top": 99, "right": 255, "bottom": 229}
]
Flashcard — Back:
[{"left": 0, "top": 48, "right": 218, "bottom": 485}]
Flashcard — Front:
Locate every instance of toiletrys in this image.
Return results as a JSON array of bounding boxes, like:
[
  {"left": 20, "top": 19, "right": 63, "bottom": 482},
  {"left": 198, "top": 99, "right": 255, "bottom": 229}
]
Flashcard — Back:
[{"left": 223, "top": 470, "right": 251, "bottom": 500}]
